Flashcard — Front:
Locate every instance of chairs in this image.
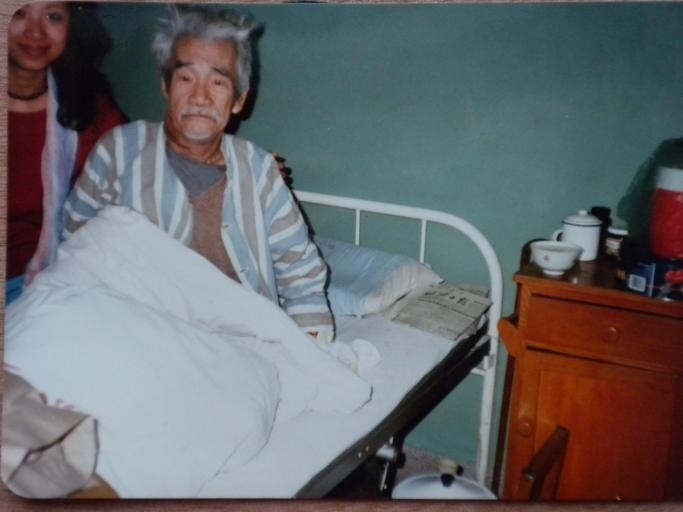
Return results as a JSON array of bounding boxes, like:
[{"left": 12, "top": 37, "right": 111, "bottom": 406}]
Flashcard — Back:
[{"left": 518, "top": 417, "right": 569, "bottom": 500}]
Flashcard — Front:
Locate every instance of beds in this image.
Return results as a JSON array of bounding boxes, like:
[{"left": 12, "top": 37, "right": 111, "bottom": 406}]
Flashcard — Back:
[{"left": 2, "top": 181, "right": 502, "bottom": 498}]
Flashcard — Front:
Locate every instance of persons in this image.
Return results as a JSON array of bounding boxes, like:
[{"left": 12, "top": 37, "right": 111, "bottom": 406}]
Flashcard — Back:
[
  {"left": 52, "top": 8, "right": 337, "bottom": 357},
  {"left": 4, "top": 1, "right": 287, "bottom": 312}
]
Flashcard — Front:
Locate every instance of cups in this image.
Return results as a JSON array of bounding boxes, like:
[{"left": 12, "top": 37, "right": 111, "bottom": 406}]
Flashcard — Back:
[
  {"left": 566, "top": 262, "right": 601, "bottom": 285},
  {"left": 552, "top": 209, "right": 602, "bottom": 261}
]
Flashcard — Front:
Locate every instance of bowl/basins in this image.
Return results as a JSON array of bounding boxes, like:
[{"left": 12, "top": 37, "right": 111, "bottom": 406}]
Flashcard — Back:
[{"left": 529, "top": 241, "right": 583, "bottom": 278}]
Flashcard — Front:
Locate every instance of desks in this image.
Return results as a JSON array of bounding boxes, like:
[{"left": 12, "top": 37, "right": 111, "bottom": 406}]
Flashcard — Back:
[{"left": 492, "top": 238, "right": 682, "bottom": 503}]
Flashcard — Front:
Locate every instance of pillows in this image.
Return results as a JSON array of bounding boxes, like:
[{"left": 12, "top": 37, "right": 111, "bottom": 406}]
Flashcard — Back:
[{"left": 316, "top": 233, "right": 441, "bottom": 315}]
